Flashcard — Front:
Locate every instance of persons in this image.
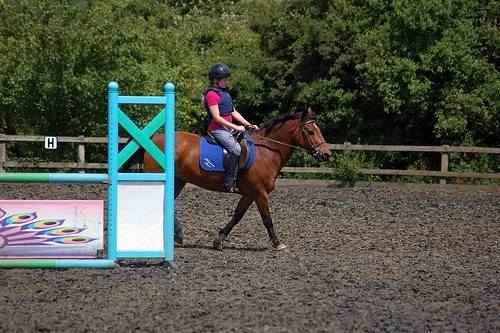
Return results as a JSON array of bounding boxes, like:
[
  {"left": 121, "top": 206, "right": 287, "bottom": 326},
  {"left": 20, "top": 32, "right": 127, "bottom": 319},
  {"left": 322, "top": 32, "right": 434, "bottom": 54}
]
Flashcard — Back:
[{"left": 204, "top": 64, "right": 259, "bottom": 193}]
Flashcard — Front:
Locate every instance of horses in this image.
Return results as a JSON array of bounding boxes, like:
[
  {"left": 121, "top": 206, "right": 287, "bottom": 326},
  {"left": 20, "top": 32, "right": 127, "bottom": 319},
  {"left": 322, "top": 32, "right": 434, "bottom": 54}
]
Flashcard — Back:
[{"left": 124, "top": 106, "right": 333, "bottom": 253}]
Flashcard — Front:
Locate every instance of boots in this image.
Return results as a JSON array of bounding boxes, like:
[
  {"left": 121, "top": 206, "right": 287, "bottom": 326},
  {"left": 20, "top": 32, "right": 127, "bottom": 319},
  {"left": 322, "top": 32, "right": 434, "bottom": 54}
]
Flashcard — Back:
[{"left": 222, "top": 151, "right": 239, "bottom": 189}]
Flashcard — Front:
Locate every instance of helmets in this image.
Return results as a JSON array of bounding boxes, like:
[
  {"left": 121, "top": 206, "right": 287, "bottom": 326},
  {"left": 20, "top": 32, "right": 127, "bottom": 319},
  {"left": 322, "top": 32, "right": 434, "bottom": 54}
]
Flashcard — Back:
[{"left": 208, "top": 63, "right": 231, "bottom": 77}]
